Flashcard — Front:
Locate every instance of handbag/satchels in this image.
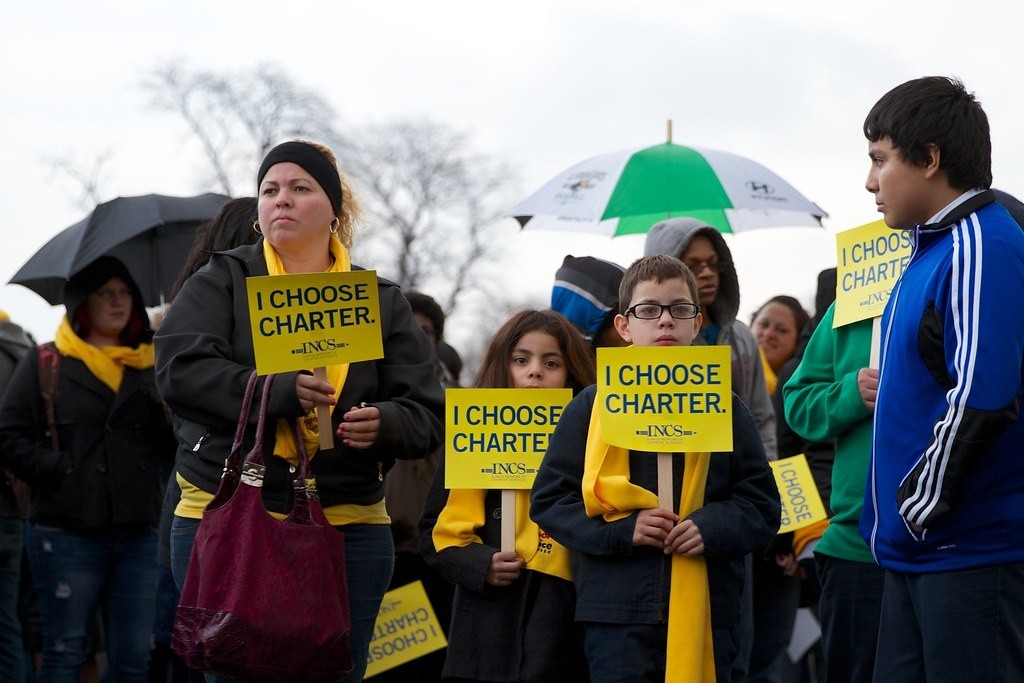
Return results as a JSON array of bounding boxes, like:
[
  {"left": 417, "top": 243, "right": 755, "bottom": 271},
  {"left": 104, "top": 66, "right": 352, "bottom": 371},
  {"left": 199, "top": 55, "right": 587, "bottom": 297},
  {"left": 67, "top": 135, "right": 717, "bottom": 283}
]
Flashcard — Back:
[{"left": 171, "top": 370, "right": 355, "bottom": 683}]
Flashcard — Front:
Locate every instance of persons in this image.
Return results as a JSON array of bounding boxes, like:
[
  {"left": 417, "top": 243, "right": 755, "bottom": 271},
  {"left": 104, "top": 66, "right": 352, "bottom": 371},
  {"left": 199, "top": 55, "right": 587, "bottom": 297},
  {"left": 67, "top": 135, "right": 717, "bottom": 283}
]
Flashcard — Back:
[
  {"left": 529, "top": 255, "right": 782, "bottom": 683},
  {"left": 864, "top": 75, "right": 1024, "bottom": 682},
  {"left": 0, "top": 197, "right": 260, "bottom": 683},
  {"left": 364, "top": 255, "right": 627, "bottom": 683},
  {"left": 153, "top": 139, "right": 446, "bottom": 683},
  {"left": 644, "top": 219, "right": 881, "bottom": 683}
]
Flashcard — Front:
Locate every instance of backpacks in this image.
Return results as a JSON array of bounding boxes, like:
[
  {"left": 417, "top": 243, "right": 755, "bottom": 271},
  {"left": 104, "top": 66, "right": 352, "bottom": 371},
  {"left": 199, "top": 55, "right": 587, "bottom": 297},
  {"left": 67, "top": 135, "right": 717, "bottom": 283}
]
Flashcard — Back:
[{"left": 7, "top": 342, "right": 59, "bottom": 520}]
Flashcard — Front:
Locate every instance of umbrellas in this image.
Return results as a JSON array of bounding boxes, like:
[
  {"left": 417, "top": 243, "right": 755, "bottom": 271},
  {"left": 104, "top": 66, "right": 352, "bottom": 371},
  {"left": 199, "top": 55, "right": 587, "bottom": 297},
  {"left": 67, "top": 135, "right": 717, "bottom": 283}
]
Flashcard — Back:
[
  {"left": 6, "top": 192, "right": 237, "bottom": 309},
  {"left": 501, "top": 119, "right": 830, "bottom": 238}
]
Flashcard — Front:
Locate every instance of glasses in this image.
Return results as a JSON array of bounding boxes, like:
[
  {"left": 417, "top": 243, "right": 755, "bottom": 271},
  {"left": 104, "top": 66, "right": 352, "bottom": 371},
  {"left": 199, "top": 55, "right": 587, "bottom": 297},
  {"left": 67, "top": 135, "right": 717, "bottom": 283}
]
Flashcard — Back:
[
  {"left": 688, "top": 263, "right": 726, "bottom": 274},
  {"left": 624, "top": 304, "right": 701, "bottom": 320}
]
